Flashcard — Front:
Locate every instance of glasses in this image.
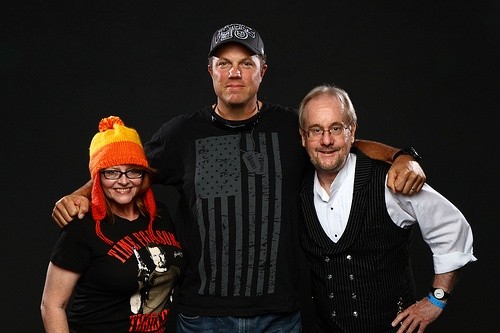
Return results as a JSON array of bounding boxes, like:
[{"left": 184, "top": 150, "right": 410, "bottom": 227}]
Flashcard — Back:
[
  {"left": 299, "top": 119, "right": 355, "bottom": 142},
  {"left": 99, "top": 168, "right": 149, "bottom": 181}
]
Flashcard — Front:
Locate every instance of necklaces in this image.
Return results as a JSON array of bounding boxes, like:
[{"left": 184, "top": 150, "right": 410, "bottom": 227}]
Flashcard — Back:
[{"left": 213, "top": 101, "right": 262, "bottom": 112}]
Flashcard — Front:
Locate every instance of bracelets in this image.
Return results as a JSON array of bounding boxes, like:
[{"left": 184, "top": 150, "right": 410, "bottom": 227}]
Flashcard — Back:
[{"left": 426, "top": 292, "right": 447, "bottom": 309}]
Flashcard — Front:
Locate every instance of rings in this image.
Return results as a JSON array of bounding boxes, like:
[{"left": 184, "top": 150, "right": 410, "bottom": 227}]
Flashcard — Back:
[{"left": 411, "top": 186, "right": 417, "bottom": 192}]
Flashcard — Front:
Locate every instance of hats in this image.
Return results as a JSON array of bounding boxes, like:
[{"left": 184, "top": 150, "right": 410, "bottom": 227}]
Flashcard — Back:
[
  {"left": 207, "top": 23, "right": 265, "bottom": 59},
  {"left": 88, "top": 116, "right": 149, "bottom": 182}
]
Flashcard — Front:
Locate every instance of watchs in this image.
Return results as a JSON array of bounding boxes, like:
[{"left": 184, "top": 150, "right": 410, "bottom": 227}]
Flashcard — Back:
[
  {"left": 430, "top": 286, "right": 450, "bottom": 300},
  {"left": 393, "top": 146, "right": 423, "bottom": 163}
]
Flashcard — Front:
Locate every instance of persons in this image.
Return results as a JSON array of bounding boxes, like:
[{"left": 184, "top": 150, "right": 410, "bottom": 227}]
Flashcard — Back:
[
  {"left": 40, "top": 115, "right": 187, "bottom": 333},
  {"left": 48, "top": 24, "right": 427, "bottom": 333},
  {"left": 298, "top": 85, "right": 479, "bottom": 333}
]
